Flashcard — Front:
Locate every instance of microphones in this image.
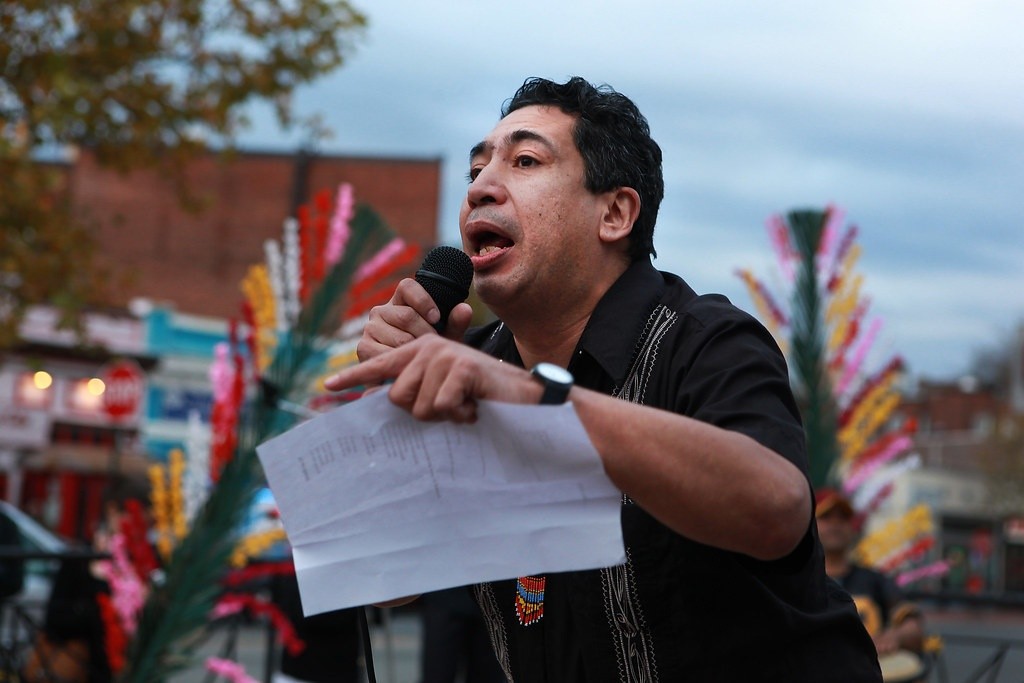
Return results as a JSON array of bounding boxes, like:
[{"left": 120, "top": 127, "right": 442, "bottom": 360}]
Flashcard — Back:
[{"left": 413, "top": 246, "right": 474, "bottom": 338}]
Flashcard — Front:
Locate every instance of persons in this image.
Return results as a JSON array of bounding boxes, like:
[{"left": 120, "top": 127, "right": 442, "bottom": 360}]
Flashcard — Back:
[
  {"left": 325, "top": 76, "right": 883, "bottom": 683},
  {"left": 16, "top": 471, "right": 172, "bottom": 683},
  {"left": 813, "top": 484, "right": 925, "bottom": 681}
]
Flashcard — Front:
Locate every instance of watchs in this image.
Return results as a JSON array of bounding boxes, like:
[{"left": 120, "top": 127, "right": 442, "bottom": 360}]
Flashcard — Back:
[{"left": 529, "top": 361, "right": 575, "bottom": 405}]
